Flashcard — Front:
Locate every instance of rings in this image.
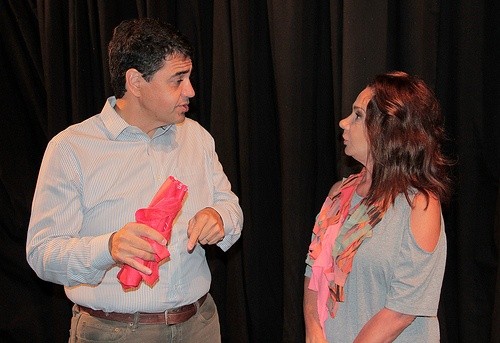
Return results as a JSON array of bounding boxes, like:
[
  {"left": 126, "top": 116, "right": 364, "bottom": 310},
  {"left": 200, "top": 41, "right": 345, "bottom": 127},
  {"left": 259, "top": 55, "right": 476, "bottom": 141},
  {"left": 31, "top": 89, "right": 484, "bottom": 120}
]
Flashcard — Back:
[{"left": 205, "top": 237, "right": 209, "bottom": 243}]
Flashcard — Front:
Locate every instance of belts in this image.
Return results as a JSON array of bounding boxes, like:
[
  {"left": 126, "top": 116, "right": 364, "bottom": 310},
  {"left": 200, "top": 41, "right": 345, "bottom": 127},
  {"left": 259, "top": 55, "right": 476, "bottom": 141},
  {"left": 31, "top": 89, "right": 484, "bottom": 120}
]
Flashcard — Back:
[{"left": 79, "top": 293, "right": 207, "bottom": 325}]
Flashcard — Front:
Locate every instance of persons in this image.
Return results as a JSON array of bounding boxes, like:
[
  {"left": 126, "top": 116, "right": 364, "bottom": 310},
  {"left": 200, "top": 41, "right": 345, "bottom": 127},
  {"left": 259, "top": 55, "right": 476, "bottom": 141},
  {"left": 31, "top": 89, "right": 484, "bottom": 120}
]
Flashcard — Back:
[
  {"left": 27, "top": 18, "right": 243, "bottom": 343},
  {"left": 303, "top": 71, "right": 460, "bottom": 343}
]
